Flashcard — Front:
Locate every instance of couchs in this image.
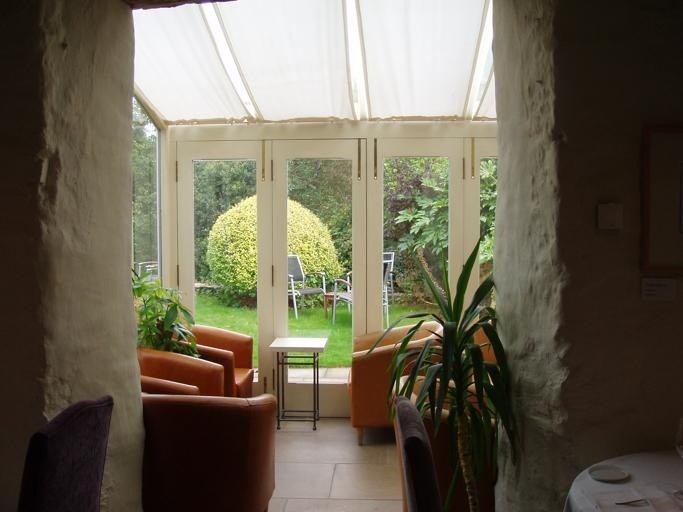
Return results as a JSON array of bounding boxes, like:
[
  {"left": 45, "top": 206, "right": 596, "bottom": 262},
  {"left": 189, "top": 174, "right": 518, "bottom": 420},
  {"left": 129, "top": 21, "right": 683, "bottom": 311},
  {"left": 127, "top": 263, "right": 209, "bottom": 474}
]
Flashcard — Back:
[
  {"left": 351, "top": 321, "right": 445, "bottom": 445},
  {"left": 392, "top": 368, "right": 497, "bottom": 427},
  {"left": 136, "top": 348, "right": 226, "bottom": 397},
  {"left": 138, "top": 324, "right": 275, "bottom": 398},
  {"left": 139, "top": 391, "right": 278, "bottom": 511}
]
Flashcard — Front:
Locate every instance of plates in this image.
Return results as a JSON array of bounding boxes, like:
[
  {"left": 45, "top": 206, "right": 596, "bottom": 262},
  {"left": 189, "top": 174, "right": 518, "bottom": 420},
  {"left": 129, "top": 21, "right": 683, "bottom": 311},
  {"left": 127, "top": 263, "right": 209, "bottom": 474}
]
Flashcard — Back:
[{"left": 588, "top": 464, "right": 630, "bottom": 481}]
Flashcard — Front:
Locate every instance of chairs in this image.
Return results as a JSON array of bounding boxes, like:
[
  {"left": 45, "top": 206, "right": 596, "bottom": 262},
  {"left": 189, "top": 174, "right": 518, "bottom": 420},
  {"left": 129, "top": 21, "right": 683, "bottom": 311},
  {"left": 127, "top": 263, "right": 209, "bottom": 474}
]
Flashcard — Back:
[
  {"left": 394, "top": 396, "right": 445, "bottom": 511},
  {"left": 137, "top": 260, "right": 158, "bottom": 277},
  {"left": 346, "top": 251, "right": 395, "bottom": 311},
  {"left": 287, "top": 255, "right": 326, "bottom": 319},
  {"left": 17, "top": 395, "right": 114, "bottom": 512},
  {"left": 329, "top": 262, "right": 390, "bottom": 328}
]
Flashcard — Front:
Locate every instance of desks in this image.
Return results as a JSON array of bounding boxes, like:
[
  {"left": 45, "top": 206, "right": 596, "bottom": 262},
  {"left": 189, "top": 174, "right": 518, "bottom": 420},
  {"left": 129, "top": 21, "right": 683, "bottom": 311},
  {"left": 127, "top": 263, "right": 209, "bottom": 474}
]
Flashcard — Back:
[
  {"left": 269, "top": 337, "right": 327, "bottom": 431},
  {"left": 567, "top": 448, "right": 682, "bottom": 511}
]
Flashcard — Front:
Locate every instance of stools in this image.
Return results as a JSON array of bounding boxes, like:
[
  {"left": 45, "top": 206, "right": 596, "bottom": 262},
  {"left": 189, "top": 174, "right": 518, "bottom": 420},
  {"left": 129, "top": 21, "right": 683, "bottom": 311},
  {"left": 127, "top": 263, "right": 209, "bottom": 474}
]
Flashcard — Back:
[{"left": 324, "top": 292, "right": 334, "bottom": 319}]
